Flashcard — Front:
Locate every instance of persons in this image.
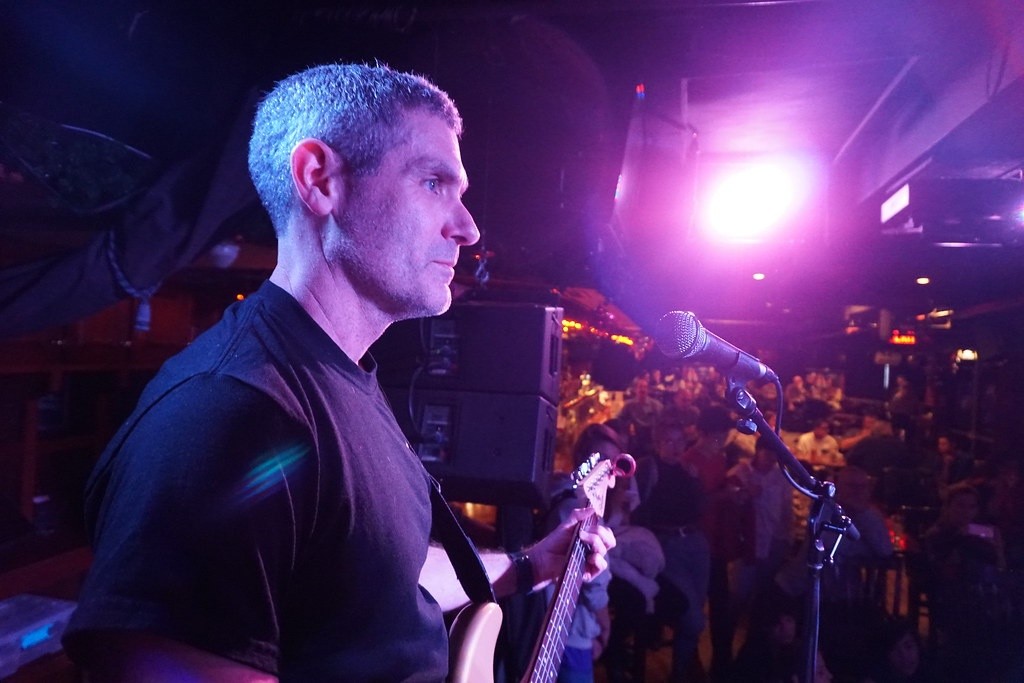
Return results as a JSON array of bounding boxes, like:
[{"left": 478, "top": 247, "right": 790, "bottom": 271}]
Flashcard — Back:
[
  {"left": 61, "top": 64, "right": 617, "bottom": 683},
  {"left": 534, "top": 367, "right": 986, "bottom": 683}
]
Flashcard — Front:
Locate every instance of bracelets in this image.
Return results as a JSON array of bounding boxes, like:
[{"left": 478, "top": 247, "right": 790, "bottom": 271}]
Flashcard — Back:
[{"left": 507, "top": 552, "right": 538, "bottom": 598}]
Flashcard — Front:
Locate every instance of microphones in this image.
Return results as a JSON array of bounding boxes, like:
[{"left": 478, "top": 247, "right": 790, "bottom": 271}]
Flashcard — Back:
[{"left": 654, "top": 311, "right": 779, "bottom": 382}]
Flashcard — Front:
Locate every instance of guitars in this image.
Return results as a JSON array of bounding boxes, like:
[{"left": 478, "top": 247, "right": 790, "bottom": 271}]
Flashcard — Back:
[{"left": 444, "top": 449, "right": 617, "bottom": 683}]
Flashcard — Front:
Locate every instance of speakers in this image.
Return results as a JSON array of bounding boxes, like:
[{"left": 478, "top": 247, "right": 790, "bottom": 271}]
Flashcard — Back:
[{"left": 413, "top": 299, "right": 565, "bottom": 513}]
